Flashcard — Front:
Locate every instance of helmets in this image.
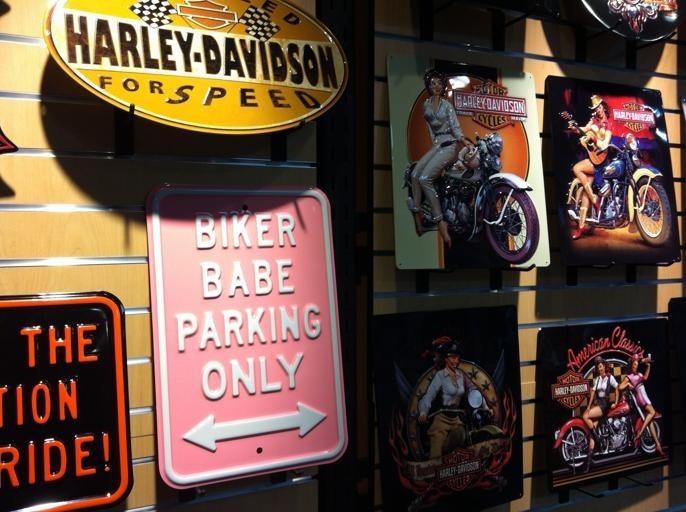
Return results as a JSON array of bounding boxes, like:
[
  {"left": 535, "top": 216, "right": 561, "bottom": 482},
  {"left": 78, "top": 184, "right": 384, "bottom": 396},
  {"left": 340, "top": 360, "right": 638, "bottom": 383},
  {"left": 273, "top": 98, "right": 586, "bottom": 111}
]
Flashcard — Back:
[{"left": 458, "top": 144, "right": 480, "bottom": 168}]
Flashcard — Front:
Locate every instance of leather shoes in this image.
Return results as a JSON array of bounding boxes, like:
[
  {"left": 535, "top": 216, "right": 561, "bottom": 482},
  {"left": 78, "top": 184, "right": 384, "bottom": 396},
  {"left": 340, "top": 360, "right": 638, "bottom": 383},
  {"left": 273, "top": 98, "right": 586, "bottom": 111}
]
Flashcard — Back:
[
  {"left": 655, "top": 446, "right": 666, "bottom": 457},
  {"left": 593, "top": 195, "right": 600, "bottom": 210},
  {"left": 634, "top": 432, "right": 640, "bottom": 442},
  {"left": 573, "top": 226, "right": 587, "bottom": 239}
]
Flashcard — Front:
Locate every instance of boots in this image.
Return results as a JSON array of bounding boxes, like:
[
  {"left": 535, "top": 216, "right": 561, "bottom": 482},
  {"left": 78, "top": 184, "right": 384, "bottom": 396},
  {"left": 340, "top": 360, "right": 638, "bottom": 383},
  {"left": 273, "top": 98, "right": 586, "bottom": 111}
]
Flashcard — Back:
[
  {"left": 590, "top": 427, "right": 600, "bottom": 451},
  {"left": 582, "top": 448, "right": 592, "bottom": 472}
]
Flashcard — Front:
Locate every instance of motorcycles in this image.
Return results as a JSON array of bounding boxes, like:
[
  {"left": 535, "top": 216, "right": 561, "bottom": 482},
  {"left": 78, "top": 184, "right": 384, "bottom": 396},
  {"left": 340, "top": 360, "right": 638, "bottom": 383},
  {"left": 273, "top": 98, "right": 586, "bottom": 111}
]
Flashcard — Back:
[
  {"left": 567, "top": 131, "right": 672, "bottom": 247},
  {"left": 554, "top": 388, "right": 661, "bottom": 468},
  {"left": 417, "top": 389, "right": 506, "bottom": 496},
  {"left": 403, "top": 132, "right": 538, "bottom": 265}
]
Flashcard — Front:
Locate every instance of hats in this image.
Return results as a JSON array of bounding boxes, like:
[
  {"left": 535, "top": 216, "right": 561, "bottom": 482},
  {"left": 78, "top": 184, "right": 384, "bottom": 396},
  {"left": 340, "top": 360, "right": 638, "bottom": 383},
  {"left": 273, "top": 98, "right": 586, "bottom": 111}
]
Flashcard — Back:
[
  {"left": 594, "top": 356, "right": 604, "bottom": 364},
  {"left": 438, "top": 343, "right": 468, "bottom": 357},
  {"left": 590, "top": 96, "right": 602, "bottom": 109}
]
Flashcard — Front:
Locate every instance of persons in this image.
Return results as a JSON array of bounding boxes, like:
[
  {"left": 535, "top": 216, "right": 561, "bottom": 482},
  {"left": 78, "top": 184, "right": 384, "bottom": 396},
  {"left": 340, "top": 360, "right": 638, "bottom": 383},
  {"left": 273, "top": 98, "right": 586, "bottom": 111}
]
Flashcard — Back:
[
  {"left": 411, "top": 70, "right": 470, "bottom": 248},
  {"left": 620, "top": 359, "right": 668, "bottom": 458},
  {"left": 581, "top": 356, "right": 620, "bottom": 473},
  {"left": 568, "top": 95, "right": 612, "bottom": 239},
  {"left": 418, "top": 343, "right": 494, "bottom": 459}
]
